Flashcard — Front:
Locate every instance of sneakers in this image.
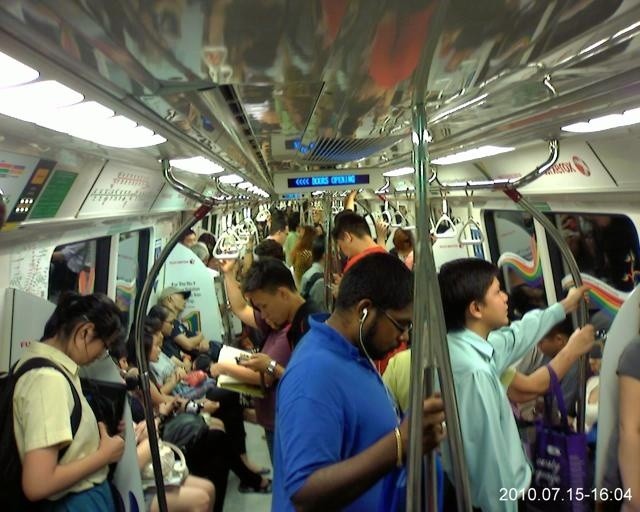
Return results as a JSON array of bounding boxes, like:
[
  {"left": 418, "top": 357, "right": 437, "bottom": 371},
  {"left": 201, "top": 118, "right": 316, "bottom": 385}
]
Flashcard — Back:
[{"left": 238, "top": 478, "right": 273, "bottom": 494}]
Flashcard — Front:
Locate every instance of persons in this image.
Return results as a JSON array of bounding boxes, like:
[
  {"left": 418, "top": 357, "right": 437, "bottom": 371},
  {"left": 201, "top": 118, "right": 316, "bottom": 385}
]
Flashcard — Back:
[
  {"left": 0, "top": 184, "right": 639, "bottom": 511},
  {"left": 200, "top": 0, "right": 521, "bottom": 141}
]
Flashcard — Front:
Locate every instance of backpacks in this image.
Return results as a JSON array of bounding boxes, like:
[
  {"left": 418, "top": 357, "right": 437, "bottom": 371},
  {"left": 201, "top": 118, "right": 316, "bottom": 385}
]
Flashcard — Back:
[{"left": 0, "top": 358, "right": 81, "bottom": 511}]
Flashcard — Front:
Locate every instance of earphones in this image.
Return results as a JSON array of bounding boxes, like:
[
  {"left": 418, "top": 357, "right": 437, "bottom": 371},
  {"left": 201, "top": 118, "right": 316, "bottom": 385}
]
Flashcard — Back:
[
  {"left": 361, "top": 308, "right": 367, "bottom": 323},
  {"left": 84, "top": 329, "right": 88, "bottom": 339}
]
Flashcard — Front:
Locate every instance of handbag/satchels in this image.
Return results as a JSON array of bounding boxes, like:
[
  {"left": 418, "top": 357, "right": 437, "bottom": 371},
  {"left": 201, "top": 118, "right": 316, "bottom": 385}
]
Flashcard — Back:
[
  {"left": 163, "top": 365, "right": 215, "bottom": 401},
  {"left": 133, "top": 422, "right": 175, "bottom": 479},
  {"left": 158, "top": 413, "right": 209, "bottom": 452},
  {"left": 536, "top": 364, "right": 592, "bottom": 512}
]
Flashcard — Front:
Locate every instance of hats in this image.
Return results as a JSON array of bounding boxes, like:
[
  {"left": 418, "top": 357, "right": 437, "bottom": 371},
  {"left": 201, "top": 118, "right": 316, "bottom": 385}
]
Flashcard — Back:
[{"left": 160, "top": 287, "right": 191, "bottom": 301}]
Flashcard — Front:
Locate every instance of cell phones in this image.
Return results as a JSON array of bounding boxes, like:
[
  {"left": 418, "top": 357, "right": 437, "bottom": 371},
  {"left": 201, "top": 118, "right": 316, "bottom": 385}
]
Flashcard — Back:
[{"left": 236, "top": 357, "right": 249, "bottom": 363}]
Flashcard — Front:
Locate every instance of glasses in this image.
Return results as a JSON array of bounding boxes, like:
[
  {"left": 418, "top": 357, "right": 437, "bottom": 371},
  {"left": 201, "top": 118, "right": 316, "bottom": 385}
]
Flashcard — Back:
[
  {"left": 164, "top": 319, "right": 175, "bottom": 325},
  {"left": 377, "top": 306, "right": 412, "bottom": 333},
  {"left": 83, "top": 314, "right": 112, "bottom": 361}
]
